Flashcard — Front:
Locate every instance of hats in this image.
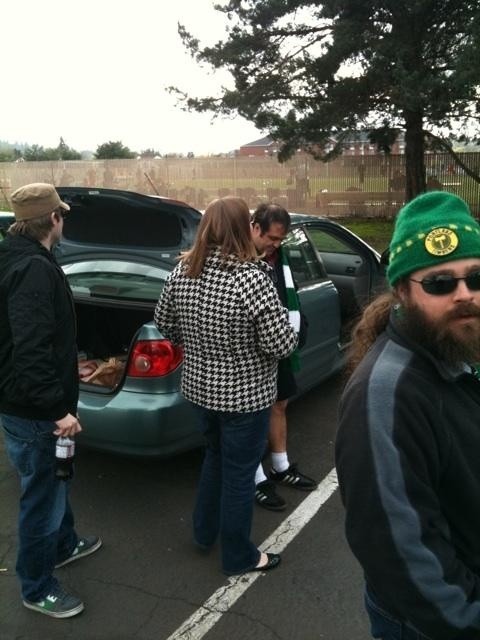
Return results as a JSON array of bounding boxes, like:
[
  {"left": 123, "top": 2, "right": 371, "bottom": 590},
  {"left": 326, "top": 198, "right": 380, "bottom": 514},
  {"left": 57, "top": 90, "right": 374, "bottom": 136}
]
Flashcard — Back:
[
  {"left": 12, "top": 184, "right": 71, "bottom": 221},
  {"left": 387, "top": 191, "right": 479, "bottom": 287}
]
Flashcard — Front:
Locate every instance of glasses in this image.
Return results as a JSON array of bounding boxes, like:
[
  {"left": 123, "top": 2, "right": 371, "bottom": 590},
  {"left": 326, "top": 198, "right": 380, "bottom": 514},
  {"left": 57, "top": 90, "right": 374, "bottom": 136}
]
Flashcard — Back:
[{"left": 408, "top": 270, "right": 479, "bottom": 295}]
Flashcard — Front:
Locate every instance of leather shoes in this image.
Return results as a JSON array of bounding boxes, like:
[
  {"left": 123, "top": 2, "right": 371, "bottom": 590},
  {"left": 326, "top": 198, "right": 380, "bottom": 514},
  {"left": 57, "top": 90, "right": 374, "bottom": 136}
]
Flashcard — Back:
[{"left": 254, "top": 553, "right": 281, "bottom": 571}]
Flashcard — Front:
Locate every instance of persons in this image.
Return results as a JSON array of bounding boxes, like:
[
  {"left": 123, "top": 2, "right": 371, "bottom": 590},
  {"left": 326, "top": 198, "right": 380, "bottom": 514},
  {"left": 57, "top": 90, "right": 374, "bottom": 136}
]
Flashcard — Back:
[
  {"left": 357, "top": 159, "right": 367, "bottom": 190},
  {"left": 1, "top": 182, "right": 104, "bottom": 620},
  {"left": 60, "top": 164, "right": 115, "bottom": 185},
  {"left": 153, "top": 195, "right": 282, "bottom": 575},
  {"left": 385, "top": 163, "right": 444, "bottom": 206},
  {"left": 249, "top": 202, "right": 318, "bottom": 510},
  {"left": 334, "top": 189, "right": 480, "bottom": 638}
]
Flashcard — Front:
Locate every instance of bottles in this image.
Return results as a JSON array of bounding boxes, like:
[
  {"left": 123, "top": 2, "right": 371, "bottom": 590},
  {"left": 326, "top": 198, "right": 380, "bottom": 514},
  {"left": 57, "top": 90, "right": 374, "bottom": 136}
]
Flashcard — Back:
[{"left": 54, "top": 435, "right": 76, "bottom": 482}]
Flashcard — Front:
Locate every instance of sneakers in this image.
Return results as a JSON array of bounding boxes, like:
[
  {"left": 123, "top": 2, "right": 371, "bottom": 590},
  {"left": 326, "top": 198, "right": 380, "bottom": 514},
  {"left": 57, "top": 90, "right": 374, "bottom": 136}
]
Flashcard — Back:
[
  {"left": 54, "top": 536, "right": 102, "bottom": 569},
  {"left": 269, "top": 464, "right": 317, "bottom": 491},
  {"left": 23, "top": 589, "right": 84, "bottom": 618},
  {"left": 254, "top": 477, "right": 287, "bottom": 510}
]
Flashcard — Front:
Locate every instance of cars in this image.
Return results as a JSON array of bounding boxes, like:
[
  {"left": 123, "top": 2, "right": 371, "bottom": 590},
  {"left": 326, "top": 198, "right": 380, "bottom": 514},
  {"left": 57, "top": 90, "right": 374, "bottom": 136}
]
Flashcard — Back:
[{"left": 0, "top": 184, "right": 392, "bottom": 461}]
